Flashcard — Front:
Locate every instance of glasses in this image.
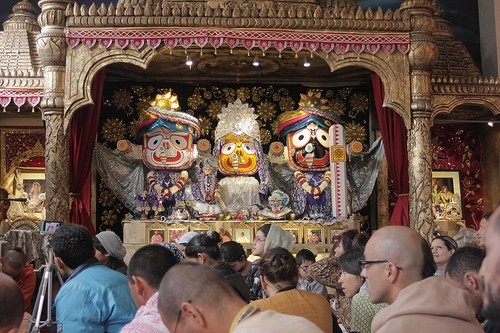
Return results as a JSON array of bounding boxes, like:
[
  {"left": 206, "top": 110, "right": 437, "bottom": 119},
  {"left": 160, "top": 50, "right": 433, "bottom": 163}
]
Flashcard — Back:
[{"left": 358, "top": 259, "right": 403, "bottom": 269}]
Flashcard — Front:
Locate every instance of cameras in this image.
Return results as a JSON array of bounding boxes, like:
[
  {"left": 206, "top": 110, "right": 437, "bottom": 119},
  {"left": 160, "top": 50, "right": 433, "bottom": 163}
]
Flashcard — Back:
[{"left": 39, "top": 221, "right": 61, "bottom": 236}]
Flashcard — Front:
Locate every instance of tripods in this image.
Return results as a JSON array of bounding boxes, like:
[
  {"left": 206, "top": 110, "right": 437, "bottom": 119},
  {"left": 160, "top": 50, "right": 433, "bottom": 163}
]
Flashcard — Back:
[{"left": 26, "top": 236, "right": 64, "bottom": 333}]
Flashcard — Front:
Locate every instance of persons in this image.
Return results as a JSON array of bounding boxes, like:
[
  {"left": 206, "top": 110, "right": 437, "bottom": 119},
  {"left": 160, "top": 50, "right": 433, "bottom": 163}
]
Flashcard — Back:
[
  {"left": 119, "top": 205, "right": 500, "bottom": 333},
  {"left": 24, "top": 182, "right": 47, "bottom": 214},
  {"left": 151, "top": 231, "right": 163, "bottom": 243},
  {"left": 0, "top": 272, "right": 25, "bottom": 333},
  {"left": 238, "top": 231, "right": 249, "bottom": 243},
  {"left": 0, "top": 249, "right": 36, "bottom": 314},
  {"left": 93, "top": 231, "right": 128, "bottom": 276},
  {"left": 332, "top": 230, "right": 342, "bottom": 243},
  {"left": 434, "top": 185, "right": 459, "bottom": 220},
  {"left": 308, "top": 231, "right": 320, "bottom": 244},
  {"left": 51, "top": 223, "right": 138, "bottom": 333}
]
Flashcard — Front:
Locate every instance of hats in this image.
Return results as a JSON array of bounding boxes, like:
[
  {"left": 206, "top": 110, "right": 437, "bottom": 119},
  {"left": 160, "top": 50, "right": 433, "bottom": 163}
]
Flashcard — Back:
[
  {"left": 178, "top": 232, "right": 199, "bottom": 243},
  {"left": 95, "top": 230, "right": 127, "bottom": 259}
]
